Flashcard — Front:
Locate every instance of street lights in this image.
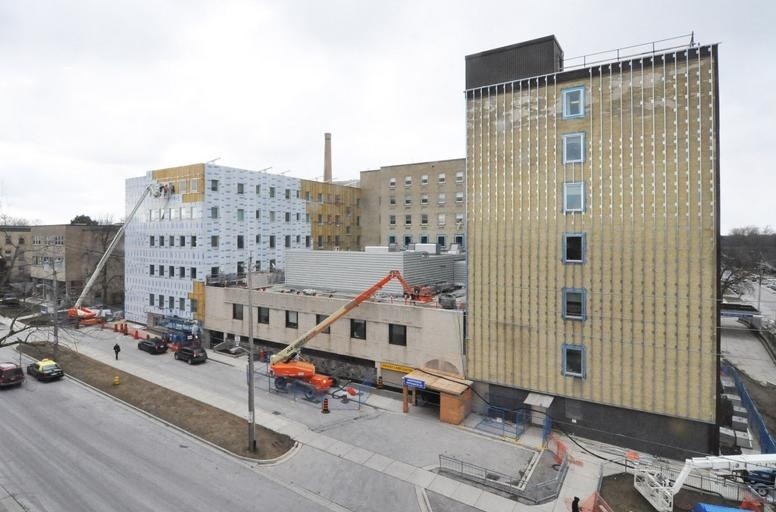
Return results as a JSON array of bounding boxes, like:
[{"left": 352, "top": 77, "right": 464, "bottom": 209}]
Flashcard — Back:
[{"left": 42, "top": 258, "right": 62, "bottom": 353}]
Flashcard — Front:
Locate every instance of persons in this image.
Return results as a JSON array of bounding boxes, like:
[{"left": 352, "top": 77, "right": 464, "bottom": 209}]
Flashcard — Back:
[
  {"left": 572, "top": 496, "right": 580, "bottom": 512},
  {"left": 113, "top": 343, "right": 120, "bottom": 360}
]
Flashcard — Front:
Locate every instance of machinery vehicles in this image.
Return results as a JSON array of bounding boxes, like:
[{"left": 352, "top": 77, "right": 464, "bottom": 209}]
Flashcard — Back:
[
  {"left": 633, "top": 453, "right": 776, "bottom": 512},
  {"left": 268, "top": 269, "right": 434, "bottom": 404},
  {"left": 66, "top": 179, "right": 174, "bottom": 329}
]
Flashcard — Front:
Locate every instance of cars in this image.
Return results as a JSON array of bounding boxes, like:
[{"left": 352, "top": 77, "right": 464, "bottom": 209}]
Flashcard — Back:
[
  {"left": 3, "top": 293, "right": 19, "bottom": 305},
  {"left": 0, "top": 362, "right": 25, "bottom": 388},
  {"left": 174, "top": 344, "right": 207, "bottom": 365},
  {"left": 138, "top": 337, "right": 169, "bottom": 355},
  {"left": 749, "top": 470, "right": 776, "bottom": 497},
  {"left": 27, "top": 358, "right": 64, "bottom": 382}
]
